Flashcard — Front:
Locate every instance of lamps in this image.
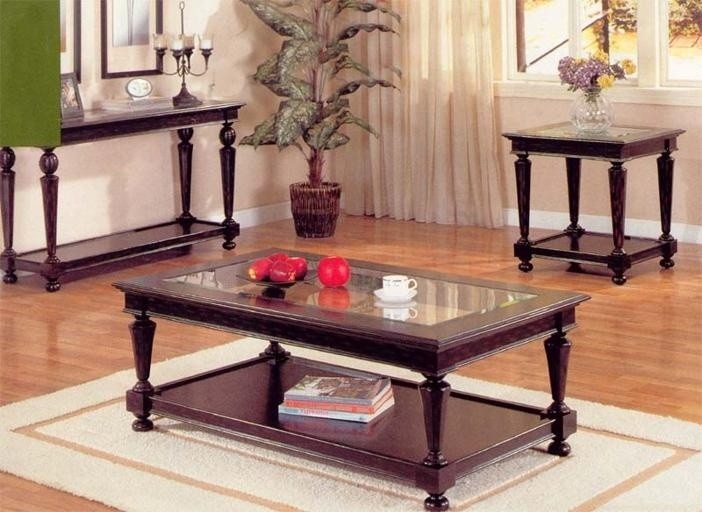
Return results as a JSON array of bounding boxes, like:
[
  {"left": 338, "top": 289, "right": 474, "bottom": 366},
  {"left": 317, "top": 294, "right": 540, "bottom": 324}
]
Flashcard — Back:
[{"left": 152, "top": 2, "right": 216, "bottom": 110}]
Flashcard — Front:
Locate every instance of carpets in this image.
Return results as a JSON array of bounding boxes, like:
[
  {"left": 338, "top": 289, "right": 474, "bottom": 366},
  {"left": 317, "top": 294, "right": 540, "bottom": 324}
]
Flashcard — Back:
[{"left": 0, "top": 337, "right": 701, "bottom": 510}]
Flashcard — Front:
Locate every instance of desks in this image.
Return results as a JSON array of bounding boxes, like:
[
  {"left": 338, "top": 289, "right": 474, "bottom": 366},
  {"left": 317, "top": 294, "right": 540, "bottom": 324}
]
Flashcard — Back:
[
  {"left": 500, "top": 121, "right": 686, "bottom": 287},
  {"left": 0, "top": 95, "right": 245, "bottom": 295}
]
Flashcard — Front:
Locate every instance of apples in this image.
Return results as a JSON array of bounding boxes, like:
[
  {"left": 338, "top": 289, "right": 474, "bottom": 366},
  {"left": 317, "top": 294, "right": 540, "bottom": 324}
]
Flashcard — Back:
[
  {"left": 319, "top": 287, "right": 350, "bottom": 309},
  {"left": 249, "top": 257, "right": 273, "bottom": 282},
  {"left": 268, "top": 261, "right": 296, "bottom": 283},
  {"left": 287, "top": 256, "right": 307, "bottom": 280},
  {"left": 317, "top": 257, "right": 351, "bottom": 288},
  {"left": 269, "top": 253, "right": 288, "bottom": 264}
]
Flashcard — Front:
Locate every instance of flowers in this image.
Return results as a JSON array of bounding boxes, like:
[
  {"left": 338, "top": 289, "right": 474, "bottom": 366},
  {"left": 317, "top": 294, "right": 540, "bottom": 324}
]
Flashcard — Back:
[{"left": 557, "top": 48, "right": 638, "bottom": 103}]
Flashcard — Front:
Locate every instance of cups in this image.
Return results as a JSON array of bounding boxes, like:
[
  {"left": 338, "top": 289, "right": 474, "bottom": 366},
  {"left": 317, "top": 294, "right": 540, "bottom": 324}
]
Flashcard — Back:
[
  {"left": 383, "top": 309, "right": 418, "bottom": 322},
  {"left": 382, "top": 274, "right": 418, "bottom": 297}
]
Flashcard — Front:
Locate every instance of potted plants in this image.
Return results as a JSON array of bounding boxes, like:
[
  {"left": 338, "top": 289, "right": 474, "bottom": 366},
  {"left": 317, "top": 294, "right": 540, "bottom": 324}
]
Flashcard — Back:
[{"left": 236, "top": 1, "right": 407, "bottom": 242}]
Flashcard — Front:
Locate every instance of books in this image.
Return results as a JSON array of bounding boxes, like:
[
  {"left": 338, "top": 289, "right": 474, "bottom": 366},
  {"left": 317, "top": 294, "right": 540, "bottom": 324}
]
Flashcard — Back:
[{"left": 278, "top": 374, "right": 396, "bottom": 424}]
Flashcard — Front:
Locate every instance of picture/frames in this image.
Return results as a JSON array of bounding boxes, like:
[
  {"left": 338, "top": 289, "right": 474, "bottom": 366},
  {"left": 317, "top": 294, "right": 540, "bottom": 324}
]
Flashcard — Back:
[
  {"left": 60, "top": 1, "right": 166, "bottom": 83},
  {"left": 57, "top": 72, "right": 85, "bottom": 124}
]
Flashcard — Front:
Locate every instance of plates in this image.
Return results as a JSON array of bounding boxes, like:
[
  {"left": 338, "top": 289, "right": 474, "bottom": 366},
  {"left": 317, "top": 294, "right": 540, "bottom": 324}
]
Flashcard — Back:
[
  {"left": 235, "top": 270, "right": 317, "bottom": 289},
  {"left": 373, "top": 302, "right": 417, "bottom": 308},
  {"left": 374, "top": 288, "right": 417, "bottom": 302}
]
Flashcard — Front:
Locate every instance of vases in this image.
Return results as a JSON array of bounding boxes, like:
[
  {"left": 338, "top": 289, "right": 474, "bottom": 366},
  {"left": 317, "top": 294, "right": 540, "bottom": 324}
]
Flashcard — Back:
[{"left": 568, "top": 88, "right": 616, "bottom": 134}]
